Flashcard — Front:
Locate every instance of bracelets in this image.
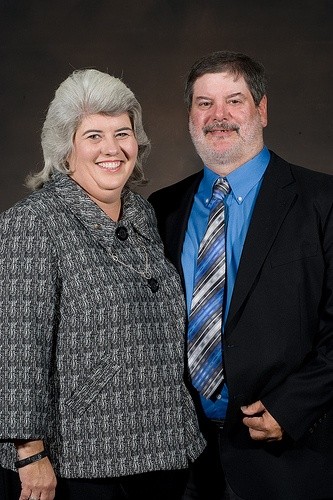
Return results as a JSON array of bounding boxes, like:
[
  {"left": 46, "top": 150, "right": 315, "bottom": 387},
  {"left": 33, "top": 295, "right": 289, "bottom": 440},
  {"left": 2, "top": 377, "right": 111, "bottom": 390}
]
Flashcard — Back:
[{"left": 15, "top": 449, "right": 49, "bottom": 468}]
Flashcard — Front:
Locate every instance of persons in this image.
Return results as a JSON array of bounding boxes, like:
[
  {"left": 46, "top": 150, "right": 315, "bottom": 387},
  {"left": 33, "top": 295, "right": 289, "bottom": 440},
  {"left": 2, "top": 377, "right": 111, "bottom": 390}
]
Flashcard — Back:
[
  {"left": 148, "top": 52, "right": 333, "bottom": 500},
  {"left": 0, "top": 68, "right": 208, "bottom": 500}
]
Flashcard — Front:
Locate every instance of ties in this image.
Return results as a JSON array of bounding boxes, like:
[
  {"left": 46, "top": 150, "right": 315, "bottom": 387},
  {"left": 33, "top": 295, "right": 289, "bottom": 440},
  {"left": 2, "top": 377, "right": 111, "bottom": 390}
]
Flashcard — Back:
[{"left": 187, "top": 177, "right": 231, "bottom": 401}]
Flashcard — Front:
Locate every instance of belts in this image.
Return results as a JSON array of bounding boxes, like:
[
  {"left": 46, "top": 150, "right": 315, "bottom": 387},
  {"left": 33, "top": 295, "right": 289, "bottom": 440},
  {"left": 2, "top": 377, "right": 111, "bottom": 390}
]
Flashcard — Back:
[{"left": 208, "top": 419, "right": 225, "bottom": 432}]
